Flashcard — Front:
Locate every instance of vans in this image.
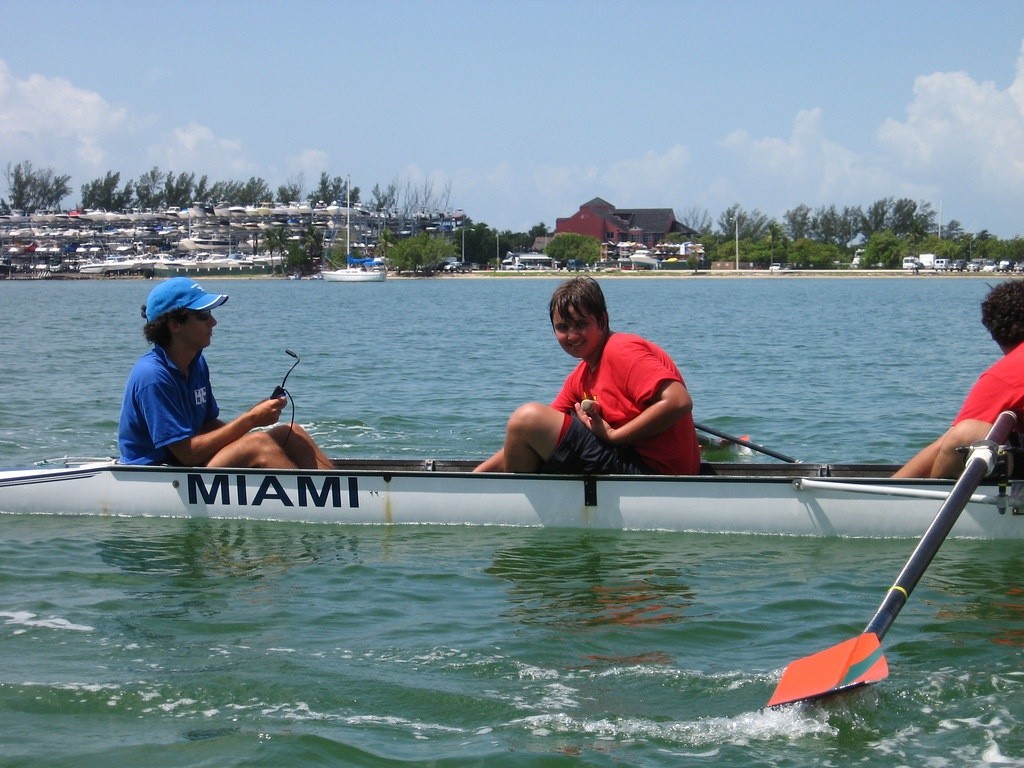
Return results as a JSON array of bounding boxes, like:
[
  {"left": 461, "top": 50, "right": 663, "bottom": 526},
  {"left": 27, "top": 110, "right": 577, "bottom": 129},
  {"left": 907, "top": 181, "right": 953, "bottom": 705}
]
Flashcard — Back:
[
  {"left": 934, "top": 259, "right": 951, "bottom": 271},
  {"left": 919, "top": 254, "right": 936, "bottom": 270},
  {"left": 502, "top": 259, "right": 525, "bottom": 270},
  {"left": 902, "top": 256, "right": 919, "bottom": 270}
]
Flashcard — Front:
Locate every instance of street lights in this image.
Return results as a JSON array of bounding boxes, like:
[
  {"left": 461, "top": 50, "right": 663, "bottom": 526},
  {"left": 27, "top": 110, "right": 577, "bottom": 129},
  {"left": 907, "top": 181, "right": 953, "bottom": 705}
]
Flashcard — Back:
[
  {"left": 462, "top": 229, "right": 480, "bottom": 261},
  {"left": 730, "top": 217, "right": 738, "bottom": 270}
]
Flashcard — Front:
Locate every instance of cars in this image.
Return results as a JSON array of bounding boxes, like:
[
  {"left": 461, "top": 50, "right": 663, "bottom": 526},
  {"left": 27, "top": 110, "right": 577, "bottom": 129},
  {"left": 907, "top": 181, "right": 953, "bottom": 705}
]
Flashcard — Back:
[
  {"left": 566, "top": 259, "right": 587, "bottom": 272},
  {"left": 953, "top": 257, "right": 1024, "bottom": 273},
  {"left": 769, "top": 262, "right": 784, "bottom": 271},
  {"left": 472, "top": 262, "right": 481, "bottom": 270}
]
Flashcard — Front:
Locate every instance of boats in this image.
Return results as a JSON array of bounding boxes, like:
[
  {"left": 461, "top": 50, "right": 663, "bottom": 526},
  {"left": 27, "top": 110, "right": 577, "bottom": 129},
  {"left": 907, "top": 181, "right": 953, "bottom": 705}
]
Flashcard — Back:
[
  {"left": 617, "top": 241, "right": 705, "bottom": 270},
  {"left": 1, "top": 433, "right": 1023, "bottom": 540},
  {"left": 0, "top": 200, "right": 463, "bottom": 275}
]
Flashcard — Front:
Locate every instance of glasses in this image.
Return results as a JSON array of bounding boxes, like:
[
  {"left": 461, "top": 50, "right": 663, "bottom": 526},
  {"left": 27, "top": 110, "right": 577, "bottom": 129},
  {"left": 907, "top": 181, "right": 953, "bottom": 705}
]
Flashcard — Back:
[{"left": 168, "top": 310, "right": 212, "bottom": 325}]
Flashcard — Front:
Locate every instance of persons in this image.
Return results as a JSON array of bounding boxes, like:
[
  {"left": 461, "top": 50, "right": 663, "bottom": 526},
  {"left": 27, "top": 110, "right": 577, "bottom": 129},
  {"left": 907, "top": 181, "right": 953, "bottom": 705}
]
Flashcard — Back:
[
  {"left": 889, "top": 279, "right": 1024, "bottom": 480},
  {"left": 471, "top": 274, "right": 700, "bottom": 475},
  {"left": 117, "top": 277, "right": 336, "bottom": 470}
]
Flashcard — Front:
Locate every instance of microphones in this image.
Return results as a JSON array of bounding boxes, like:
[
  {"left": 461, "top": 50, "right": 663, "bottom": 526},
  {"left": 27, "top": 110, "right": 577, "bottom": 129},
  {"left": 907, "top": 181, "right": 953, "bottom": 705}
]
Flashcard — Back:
[{"left": 282, "top": 349, "right": 300, "bottom": 387}]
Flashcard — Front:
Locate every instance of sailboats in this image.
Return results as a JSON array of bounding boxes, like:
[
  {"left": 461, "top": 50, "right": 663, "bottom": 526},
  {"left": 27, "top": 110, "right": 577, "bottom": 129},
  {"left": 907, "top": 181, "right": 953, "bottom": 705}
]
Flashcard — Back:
[{"left": 320, "top": 173, "right": 387, "bottom": 282}]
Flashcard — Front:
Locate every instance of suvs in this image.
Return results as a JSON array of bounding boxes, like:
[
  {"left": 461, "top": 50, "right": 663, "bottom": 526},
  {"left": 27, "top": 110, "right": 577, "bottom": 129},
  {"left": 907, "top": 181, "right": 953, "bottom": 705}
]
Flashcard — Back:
[{"left": 444, "top": 261, "right": 473, "bottom": 274}]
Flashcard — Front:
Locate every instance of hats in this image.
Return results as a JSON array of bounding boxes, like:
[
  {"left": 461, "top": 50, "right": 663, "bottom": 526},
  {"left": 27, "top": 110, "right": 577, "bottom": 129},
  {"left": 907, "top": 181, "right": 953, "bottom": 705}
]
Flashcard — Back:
[{"left": 145, "top": 276, "right": 229, "bottom": 325}]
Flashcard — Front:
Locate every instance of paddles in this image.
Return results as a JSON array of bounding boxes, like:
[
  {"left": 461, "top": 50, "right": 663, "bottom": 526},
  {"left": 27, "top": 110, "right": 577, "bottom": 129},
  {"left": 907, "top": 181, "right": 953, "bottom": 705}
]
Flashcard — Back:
[
  {"left": 764, "top": 409, "right": 1020, "bottom": 708},
  {"left": 580, "top": 398, "right": 750, "bottom": 450}
]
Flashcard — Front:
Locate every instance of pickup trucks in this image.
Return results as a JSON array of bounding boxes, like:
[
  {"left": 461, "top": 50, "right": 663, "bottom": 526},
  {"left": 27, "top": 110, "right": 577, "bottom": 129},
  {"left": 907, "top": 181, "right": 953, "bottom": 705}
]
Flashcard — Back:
[{"left": 526, "top": 263, "right": 551, "bottom": 270}]
